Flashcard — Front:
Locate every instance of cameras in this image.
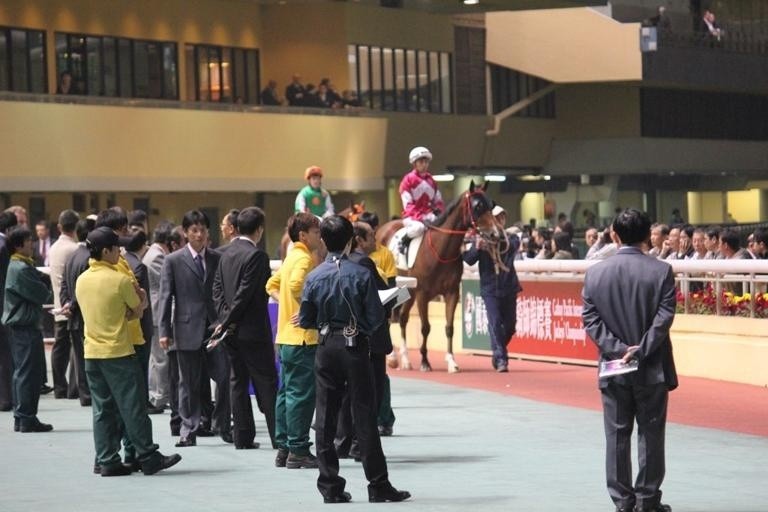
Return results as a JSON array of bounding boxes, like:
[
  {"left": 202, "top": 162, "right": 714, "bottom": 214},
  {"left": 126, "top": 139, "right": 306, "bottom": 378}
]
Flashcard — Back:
[{"left": 538, "top": 240, "right": 551, "bottom": 250}]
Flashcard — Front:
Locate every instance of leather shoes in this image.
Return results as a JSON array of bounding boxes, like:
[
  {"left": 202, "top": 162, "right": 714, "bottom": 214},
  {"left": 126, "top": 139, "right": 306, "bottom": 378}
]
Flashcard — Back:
[
  {"left": 0, "top": 400, "right": 13, "bottom": 412},
  {"left": 144, "top": 400, "right": 165, "bottom": 415},
  {"left": 169, "top": 415, "right": 262, "bottom": 451},
  {"left": 13, "top": 414, "right": 54, "bottom": 434},
  {"left": 322, "top": 490, "right": 353, "bottom": 504},
  {"left": 94, "top": 443, "right": 183, "bottom": 479},
  {"left": 331, "top": 435, "right": 366, "bottom": 461},
  {"left": 367, "top": 483, "right": 412, "bottom": 503},
  {"left": 375, "top": 423, "right": 394, "bottom": 437},
  {"left": 274, "top": 445, "right": 323, "bottom": 471},
  {"left": 38, "top": 383, "right": 93, "bottom": 408},
  {"left": 615, "top": 503, "right": 673, "bottom": 512},
  {"left": 492, "top": 357, "right": 510, "bottom": 373}
]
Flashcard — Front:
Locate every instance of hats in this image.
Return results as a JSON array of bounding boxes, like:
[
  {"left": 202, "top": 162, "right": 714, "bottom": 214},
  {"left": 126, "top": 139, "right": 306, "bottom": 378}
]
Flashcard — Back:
[
  {"left": 491, "top": 205, "right": 507, "bottom": 217},
  {"left": 86, "top": 225, "right": 134, "bottom": 253}
]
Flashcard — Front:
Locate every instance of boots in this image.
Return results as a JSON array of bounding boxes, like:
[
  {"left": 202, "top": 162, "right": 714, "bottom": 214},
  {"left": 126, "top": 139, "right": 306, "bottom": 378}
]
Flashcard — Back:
[{"left": 397, "top": 233, "right": 412, "bottom": 257}]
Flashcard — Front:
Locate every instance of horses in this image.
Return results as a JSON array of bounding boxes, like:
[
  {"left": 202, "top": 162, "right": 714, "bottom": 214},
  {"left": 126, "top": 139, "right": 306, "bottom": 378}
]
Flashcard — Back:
[
  {"left": 279, "top": 199, "right": 367, "bottom": 265},
  {"left": 374, "top": 179, "right": 501, "bottom": 374}
]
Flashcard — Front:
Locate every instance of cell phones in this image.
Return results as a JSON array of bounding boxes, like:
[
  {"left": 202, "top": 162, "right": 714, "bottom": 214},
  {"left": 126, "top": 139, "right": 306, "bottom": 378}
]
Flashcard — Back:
[{"left": 684, "top": 238, "right": 690, "bottom": 243}]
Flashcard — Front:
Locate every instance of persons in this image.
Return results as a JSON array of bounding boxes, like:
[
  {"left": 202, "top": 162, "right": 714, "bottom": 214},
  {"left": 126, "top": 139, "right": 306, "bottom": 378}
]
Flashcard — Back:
[
  {"left": 582, "top": 211, "right": 679, "bottom": 512},
  {"left": 507, "top": 209, "right": 767, "bottom": 307},
  {"left": 56, "top": 70, "right": 81, "bottom": 104},
  {"left": 397, "top": 147, "right": 445, "bottom": 253},
  {"left": 700, "top": 10, "right": 720, "bottom": 48},
  {"left": 294, "top": 166, "right": 335, "bottom": 219},
  {"left": 462, "top": 206, "right": 523, "bottom": 373},
  {"left": 0, "top": 205, "right": 412, "bottom": 505},
  {"left": 648, "top": 6, "right": 671, "bottom": 44},
  {"left": 262, "top": 73, "right": 360, "bottom": 116},
  {"left": 235, "top": 96, "right": 244, "bottom": 112}
]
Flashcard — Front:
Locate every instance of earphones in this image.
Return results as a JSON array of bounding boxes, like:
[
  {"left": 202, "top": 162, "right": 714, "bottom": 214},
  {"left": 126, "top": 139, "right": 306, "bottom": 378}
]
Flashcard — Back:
[
  {"left": 336, "top": 260, "right": 340, "bottom": 268},
  {"left": 333, "top": 256, "right": 337, "bottom": 261}
]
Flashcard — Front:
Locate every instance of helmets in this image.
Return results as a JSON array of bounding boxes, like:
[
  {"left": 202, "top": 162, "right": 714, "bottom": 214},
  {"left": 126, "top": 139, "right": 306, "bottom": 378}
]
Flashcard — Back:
[
  {"left": 303, "top": 165, "right": 325, "bottom": 181},
  {"left": 406, "top": 144, "right": 434, "bottom": 165}
]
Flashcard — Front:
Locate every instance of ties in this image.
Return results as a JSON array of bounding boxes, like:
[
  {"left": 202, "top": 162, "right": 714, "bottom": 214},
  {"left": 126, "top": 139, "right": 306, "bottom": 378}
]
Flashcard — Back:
[
  {"left": 40, "top": 237, "right": 47, "bottom": 261},
  {"left": 194, "top": 254, "right": 206, "bottom": 282}
]
